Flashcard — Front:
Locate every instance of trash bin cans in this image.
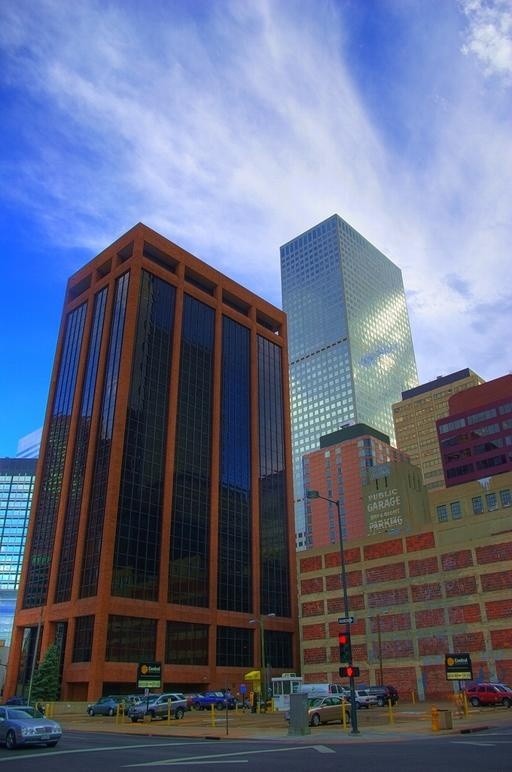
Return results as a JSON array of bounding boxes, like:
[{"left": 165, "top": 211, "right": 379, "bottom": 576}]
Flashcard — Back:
[{"left": 437, "top": 710, "right": 452, "bottom": 730}]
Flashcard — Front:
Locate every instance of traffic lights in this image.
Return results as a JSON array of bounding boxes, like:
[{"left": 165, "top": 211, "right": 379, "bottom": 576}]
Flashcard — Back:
[
  {"left": 339, "top": 667, "right": 359, "bottom": 677},
  {"left": 339, "top": 632, "right": 351, "bottom": 663}
]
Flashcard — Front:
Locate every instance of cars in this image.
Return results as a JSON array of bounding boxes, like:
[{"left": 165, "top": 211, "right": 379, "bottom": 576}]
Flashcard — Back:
[
  {"left": 343, "top": 687, "right": 399, "bottom": 709},
  {"left": 87, "top": 691, "right": 265, "bottom": 722},
  {"left": 466, "top": 683, "right": 512, "bottom": 706},
  {"left": 284, "top": 696, "right": 351, "bottom": 726},
  {"left": 0, "top": 697, "right": 62, "bottom": 750}
]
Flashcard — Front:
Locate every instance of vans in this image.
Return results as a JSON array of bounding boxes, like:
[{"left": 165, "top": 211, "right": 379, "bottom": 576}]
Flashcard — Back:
[{"left": 303, "top": 684, "right": 343, "bottom": 704}]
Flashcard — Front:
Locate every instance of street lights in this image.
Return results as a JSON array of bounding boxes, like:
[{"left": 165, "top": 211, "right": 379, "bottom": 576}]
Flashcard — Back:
[
  {"left": 249, "top": 613, "right": 276, "bottom": 704},
  {"left": 307, "top": 490, "right": 360, "bottom": 733}
]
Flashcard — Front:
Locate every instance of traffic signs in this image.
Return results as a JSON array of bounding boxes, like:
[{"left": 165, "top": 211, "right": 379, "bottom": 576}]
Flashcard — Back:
[{"left": 338, "top": 617, "right": 353, "bottom": 624}]
